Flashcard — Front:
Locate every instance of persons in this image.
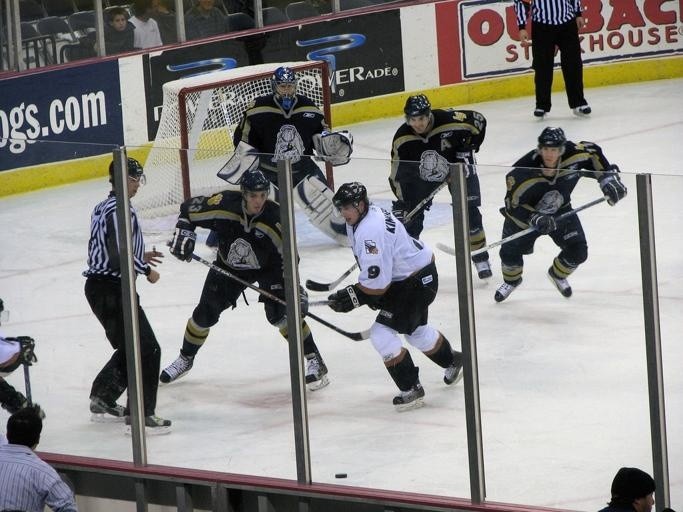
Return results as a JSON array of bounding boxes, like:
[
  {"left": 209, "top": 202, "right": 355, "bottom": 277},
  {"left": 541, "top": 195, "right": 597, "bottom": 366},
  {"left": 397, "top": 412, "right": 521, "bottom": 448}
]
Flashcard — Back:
[
  {"left": 127, "top": 0, "right": 163, "bottom": 50},
  {"left": 159, "top": 170, "right": 331, "bottom": 391},
  {"left": 184, "top": 0, "right": 229, "bottom": 41},
  {"left": 328, "top": 180, "right": 464, "bottom": 413},
  {"left": 86, "top": 7, "right": 135, "bottom": 57},
  {"left": 216, "top": 66, "right": 354, "bottom": 248},
  {"left": 0, "top": 408, "right": 78, "bottom": 512},
  {"left": 599, "top": 467, "right": 656, "bottom": 512},
  {"left": 0, "top": 298, "right": 45, "bottom": 417},
  {"left": 494, "top": 127, "right": 628, "bottom": 306},
  {"left": 515, "top": 1, "right": 593, "bottom": 121},
  {"left": 389, "top": 94, "right": 492, "bottom": 288},
  {"left": 82, "top": 157, "right": 173, "bottom": 438},
  {"left": 155, "top": 0, "right": 188, "bottom": 43}
]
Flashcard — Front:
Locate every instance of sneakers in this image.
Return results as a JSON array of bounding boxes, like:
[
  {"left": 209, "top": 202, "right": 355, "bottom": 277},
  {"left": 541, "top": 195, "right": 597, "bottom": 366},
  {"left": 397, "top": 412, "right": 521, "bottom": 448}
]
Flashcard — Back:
[
  {"left": 304, "top": 352, "right": 329, "bottom": 383},
  {"left": 160, "top": 349, "right": 194, "bottom": 384},
  {"left": 532, "top": 106, "right": 546, "bottom": 117},
  {"left": 89, "top": 397, "right": 130, "bottom": 418},
  {"left": 547, "top": 265, "right": 572, "bottom": 298},
  {"left": 392, "top": 381, "right": 425, "bottom": 406},
  {"left": 124, "top": 412, "right": 172, "bottom": 429},
  {"left": 573, "top": 103, "right": 592, "bottom": 114},
  {"left": 442, "top": 350, "right": 464, "bottom": 385},
  {"left": 494, "top": 276, "right": 523, "bottom": 303},
  {"left": 474, "top": 260, "right": 493, "bottom": 279}
]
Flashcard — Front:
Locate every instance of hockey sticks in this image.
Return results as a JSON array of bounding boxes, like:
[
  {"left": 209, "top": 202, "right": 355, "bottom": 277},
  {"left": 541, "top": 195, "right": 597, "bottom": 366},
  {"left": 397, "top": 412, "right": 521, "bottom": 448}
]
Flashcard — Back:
[
  {"left": 305, "top": 180, "right": 449, "bottom": 292},
  {"left": 437, "top": 196, "right": 606, "bottom": 255},
  {"left": 191, "top": 253, "right": 372, "bottom": 341}
]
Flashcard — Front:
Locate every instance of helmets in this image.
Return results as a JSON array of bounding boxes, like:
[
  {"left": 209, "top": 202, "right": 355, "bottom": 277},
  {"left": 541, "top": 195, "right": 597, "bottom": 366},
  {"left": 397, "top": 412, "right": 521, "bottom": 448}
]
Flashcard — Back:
[
  {"left": 537, "top": 126, "right": 568, "bottom": 148},
  {"left": 331, "top": 181, "right": 367, "bottom": 207},
  {"left": 271, "top": 66, "right": 298, "bottom": 99},
  {"left": 239, "top": 168, "right": 271, "bottom": 192},
  {"left": 403, "top": 93, "right": 432, "bottom": 116},
  {"left": 107, "top": 156, "right": 148, "bottom": 188}
]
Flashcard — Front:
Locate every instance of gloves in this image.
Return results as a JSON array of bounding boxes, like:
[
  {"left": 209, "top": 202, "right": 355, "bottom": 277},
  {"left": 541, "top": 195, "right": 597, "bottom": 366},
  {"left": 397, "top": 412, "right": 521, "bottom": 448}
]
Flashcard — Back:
[
  {"left": 528, "top": 212, "right": 557, "bottom": 233},
  {"left": 596, "top": 172, "right": 629, "bottom": 207},
  {"left": 297, "top": 283, "right": 309, "bottom": 319},
  {"left": 327, "top": 283, "right": 366, "bottom": 314},
  {"left": 455, "top": 151, "right": 476, "bottom": 179},
  {"left": 166, "top": 219, "right": 198, "bottom": 264}
]
